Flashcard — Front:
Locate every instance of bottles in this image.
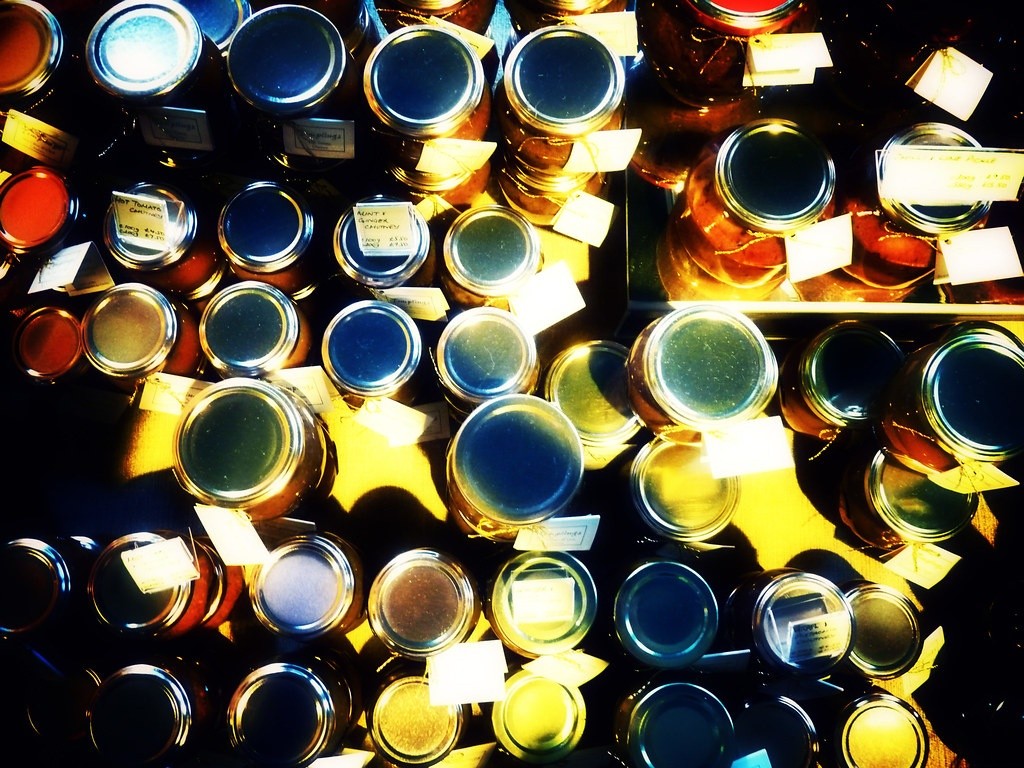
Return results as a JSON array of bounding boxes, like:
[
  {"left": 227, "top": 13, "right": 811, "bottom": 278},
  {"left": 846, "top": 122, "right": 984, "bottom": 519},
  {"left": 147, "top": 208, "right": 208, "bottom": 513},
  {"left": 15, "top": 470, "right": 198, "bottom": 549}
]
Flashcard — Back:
[{"left": 0, "top": 0, "right": 1024, "bottom": 768}]
